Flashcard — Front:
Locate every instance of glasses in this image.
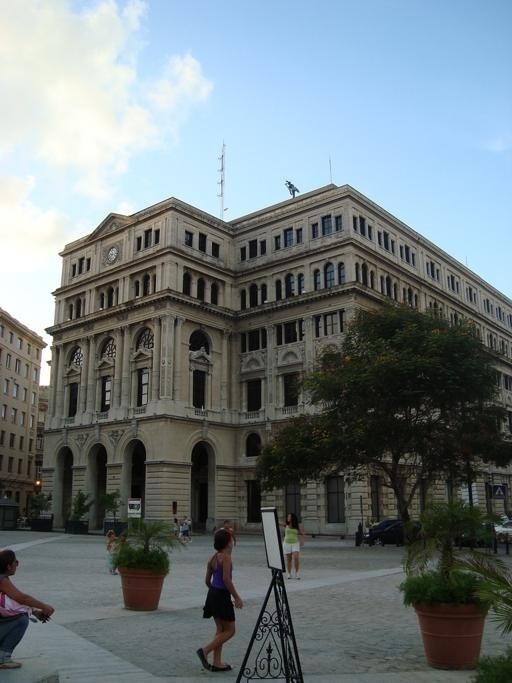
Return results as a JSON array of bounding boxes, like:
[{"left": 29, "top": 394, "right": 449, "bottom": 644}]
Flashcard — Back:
[{"left": 13, "top": 560, "right": 19, "bottom": 566}]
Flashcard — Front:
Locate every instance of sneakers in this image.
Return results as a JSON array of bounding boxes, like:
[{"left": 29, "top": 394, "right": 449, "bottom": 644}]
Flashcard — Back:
[
  {"left": 287, "top": 574, "right": 301, "bottom": 579},
  {"left": 197, "top": 648, "right": 211, "bottom": 668},
  {"left": 212, "top": 665, "right": 232, "bottom": 672},
  {"left": 1, "top": 661, "right": 22, "bottom": 669}
]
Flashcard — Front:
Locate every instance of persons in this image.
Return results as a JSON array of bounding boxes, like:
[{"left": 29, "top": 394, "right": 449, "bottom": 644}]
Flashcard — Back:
[
  {"left": 220, "top": 520, "right": 236, "bottom": 548},
  {"left": 196, "top": 530, "right": 243, "bottom": 672},
  {"left": 105, "top": 530, "right": 120, "bottom": 575},
  {"left": 173, "top": 515, "right": 193, "bottom": 543},
  {"left": 278, "top": 512, "right": 304, "bottom": 580},
  {"left": 0, "top": 550, "right": 55, "bottom": 669}
]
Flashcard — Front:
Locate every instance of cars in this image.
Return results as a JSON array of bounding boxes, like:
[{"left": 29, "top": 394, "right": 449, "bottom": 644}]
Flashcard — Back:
[
  {"left": 483, "top": 515, "right": 512, "bottom": 544},
  {"left": 363, "top": 519, "right": 421, "bottom": 547}
]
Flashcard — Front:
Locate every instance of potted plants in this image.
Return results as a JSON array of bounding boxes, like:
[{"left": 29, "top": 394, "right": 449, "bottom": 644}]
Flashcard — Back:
[
  {"left": 105, "top": 516, "right": 187, "bottom": 611},
  {"left": 398, "top": 498, "right": 507, "bottom": 671}
]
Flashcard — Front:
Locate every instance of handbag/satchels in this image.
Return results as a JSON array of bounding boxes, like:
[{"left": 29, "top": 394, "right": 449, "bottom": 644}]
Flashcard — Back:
[{"left": 0, "top": 605, "right": 38, "bottom": 623}]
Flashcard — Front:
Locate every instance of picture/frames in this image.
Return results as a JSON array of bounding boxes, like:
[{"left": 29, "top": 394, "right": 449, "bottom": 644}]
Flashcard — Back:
[{"left": 260, "top": 508, "right": 286, "bottom": 572}]
